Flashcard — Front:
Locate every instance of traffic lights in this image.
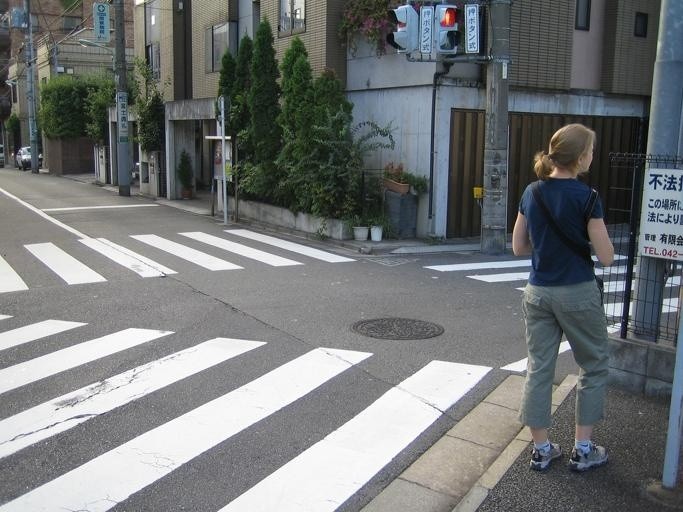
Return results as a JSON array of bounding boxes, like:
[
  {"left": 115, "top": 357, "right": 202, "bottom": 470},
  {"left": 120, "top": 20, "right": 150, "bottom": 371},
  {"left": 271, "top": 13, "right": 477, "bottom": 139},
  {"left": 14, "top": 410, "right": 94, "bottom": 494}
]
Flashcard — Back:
[
  {"left": 433, "top": 4, "right": 461, "bottom": 56},
  {"left": 388, "top": 6, "right": 419, "bottom": 53}
]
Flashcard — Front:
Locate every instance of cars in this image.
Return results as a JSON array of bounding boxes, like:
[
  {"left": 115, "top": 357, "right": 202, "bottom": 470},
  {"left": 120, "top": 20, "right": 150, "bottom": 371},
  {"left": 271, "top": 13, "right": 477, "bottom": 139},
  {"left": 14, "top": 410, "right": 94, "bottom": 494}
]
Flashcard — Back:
[{"left": 15, "top": 145, "right": 41, "bottom": 169}]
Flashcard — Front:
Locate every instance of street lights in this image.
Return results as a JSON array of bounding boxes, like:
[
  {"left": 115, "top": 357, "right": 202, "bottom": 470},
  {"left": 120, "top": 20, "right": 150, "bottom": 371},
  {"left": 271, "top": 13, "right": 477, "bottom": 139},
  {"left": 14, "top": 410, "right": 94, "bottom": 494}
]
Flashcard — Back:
[
  {"left": 4, "top": 79, "right": 38, "bottom": 172},
  {"left": 77, "top": 37, "right": 130, "bottom": 195}
]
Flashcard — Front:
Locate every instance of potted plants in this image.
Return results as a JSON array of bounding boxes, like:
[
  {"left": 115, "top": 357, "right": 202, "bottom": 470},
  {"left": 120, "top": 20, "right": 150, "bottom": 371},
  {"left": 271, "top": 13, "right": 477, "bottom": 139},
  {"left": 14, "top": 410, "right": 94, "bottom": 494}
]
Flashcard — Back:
[
  {"left": 380, "top": 162, "right": 410, "bottom": 193},
  {"left": 175, "top": 149, "right": 195, "bottom": 200},
  {"left": 347, "top": 209, "right": 392, "bottom": 242}
]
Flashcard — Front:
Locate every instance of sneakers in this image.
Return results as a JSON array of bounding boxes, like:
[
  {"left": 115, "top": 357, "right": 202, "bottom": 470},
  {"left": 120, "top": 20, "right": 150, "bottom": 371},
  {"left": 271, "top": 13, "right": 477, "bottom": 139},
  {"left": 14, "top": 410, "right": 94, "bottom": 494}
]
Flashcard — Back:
[
  {"left": 529, "top": 442, "right": 562, "bottom": 471},
  {"left": 567, "top": 443, "right": 609, "bottom": 472}
]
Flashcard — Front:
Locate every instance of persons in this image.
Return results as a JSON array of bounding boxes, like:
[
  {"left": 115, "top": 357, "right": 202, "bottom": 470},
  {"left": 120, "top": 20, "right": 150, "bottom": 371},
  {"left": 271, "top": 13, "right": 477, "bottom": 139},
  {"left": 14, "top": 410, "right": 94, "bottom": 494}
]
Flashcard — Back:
[{"left": 510, "top": 124, "right": 614, "bottom": 473}]
